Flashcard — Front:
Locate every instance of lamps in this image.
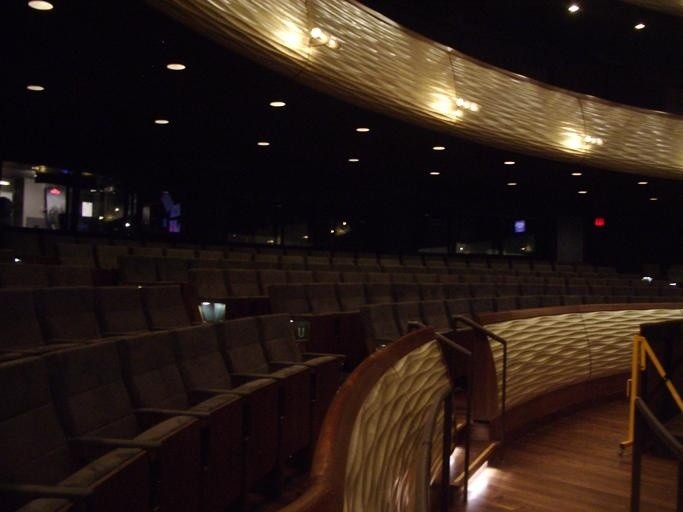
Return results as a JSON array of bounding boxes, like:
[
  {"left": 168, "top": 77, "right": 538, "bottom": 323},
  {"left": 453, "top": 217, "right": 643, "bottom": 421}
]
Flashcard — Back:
[{"left": 309, "top": 26, "right": 343, "bottom": 54}]
